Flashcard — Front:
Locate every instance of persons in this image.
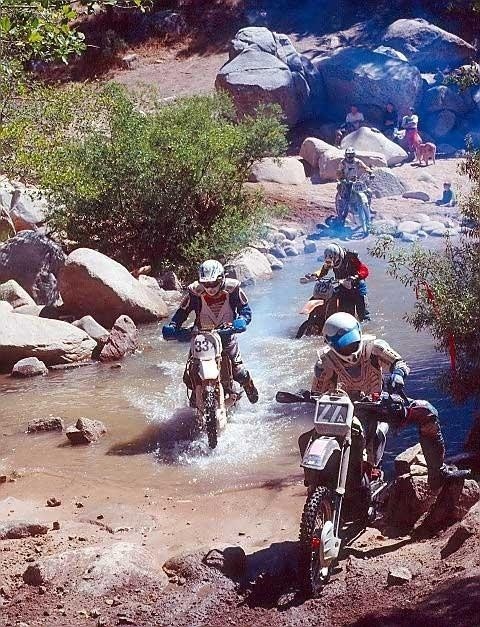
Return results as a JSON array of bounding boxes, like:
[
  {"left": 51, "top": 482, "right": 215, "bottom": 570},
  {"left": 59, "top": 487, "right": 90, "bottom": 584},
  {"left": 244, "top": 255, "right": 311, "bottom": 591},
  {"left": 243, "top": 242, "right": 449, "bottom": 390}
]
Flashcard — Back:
[
  {"left": 383, "top": 102, "right": 400, "bottom": 141},
  {"left": 305, "top": 242, "right": 370, "bottom": 322},
  {"left": 401, "top": 107, "right": 423, "bottom": 161},
  {"left": 161, "top": 259, "right": 260, "bottom": 404},
  {"left": 335, "top": 146, "right": 378, "bottom": 214},
  {"left": 436, "top": 181, "right": 456, "bottom": 208},
  {"left": 310, "top": 310, "right": 472, "bottom": 489},
  {"left": 345, "top": 104, "right": 364, "bottom": 134}
]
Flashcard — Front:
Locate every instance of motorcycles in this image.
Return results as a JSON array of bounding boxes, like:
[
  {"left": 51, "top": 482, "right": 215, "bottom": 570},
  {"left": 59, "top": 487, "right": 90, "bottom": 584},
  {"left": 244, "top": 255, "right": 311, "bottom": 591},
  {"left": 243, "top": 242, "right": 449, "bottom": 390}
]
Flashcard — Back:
[
  {"left": 276, "top": 390, "right": 389, "bottom": 598},
  {"left": 163, "top": 322, "right": 244, "bottom": 450},
  {"left": 294, "top": 273, "right": 366, "bottom": 339},
  {"left": 331, "top": 172, "right": 376, "bottom": 236}
]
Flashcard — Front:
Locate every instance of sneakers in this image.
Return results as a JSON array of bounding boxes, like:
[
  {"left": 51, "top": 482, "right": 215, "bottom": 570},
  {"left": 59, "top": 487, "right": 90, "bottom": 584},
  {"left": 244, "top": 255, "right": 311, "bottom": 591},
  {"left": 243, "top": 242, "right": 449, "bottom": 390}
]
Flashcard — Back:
[
  {"left": 243, "top": 380, "right": 258, "bottom": 405},
  {"left": 429, "top": 470, "right": 472, "bottom": 496}
]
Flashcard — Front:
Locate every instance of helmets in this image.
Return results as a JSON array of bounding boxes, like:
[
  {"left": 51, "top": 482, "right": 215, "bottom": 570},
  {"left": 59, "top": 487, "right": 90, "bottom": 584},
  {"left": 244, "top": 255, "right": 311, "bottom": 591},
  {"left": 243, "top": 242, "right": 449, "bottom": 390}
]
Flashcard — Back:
[
  {"left": 323, "top": 312, "right": 369, "bottom": 365},
  {"left": 344, "top": 147, "right": 356, "bottom": 163},
  {"left": 323, "top": 243, "right": 346, "bottom": 271},
  {"left": 197, "top": 258, "right": 225, "bottom": 297}
]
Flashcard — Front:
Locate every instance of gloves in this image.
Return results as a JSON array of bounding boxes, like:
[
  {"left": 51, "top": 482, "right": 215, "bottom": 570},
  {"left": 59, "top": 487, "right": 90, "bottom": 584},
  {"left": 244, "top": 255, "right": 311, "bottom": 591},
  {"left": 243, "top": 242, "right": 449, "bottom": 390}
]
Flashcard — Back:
[
  {"left": 233, "top": 318, "right": 246, "bottom": 332},
  {"left": 387, "top": 368, "right": 406, "bottom": 395},
  {"left": 162, "top": 322, "right": 176, "bottom": 337}
]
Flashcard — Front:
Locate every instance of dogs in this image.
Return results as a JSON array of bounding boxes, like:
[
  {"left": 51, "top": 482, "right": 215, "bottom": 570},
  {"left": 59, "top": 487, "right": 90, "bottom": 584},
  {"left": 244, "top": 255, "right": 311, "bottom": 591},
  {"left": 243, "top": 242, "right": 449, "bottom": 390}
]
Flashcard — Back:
[{"left": 412, "top": 140, "right": 436, "bottom": 166}]
